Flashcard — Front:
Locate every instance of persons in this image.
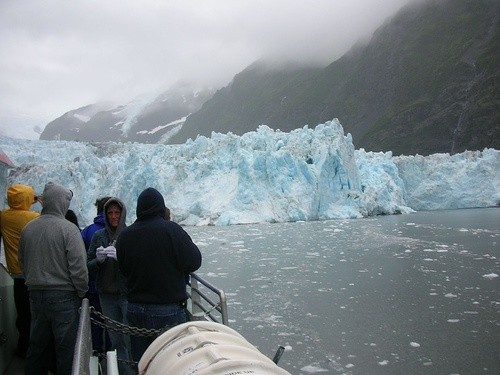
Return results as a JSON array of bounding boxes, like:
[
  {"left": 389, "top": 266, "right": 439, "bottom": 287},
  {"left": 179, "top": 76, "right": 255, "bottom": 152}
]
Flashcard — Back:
[
  {"left": 115, "top": 187, "right": 202, "bottom": 375},
  {"left": 65, "top": 197, "right": 135, "bottom": 375},
  {"left": 0, "top": 184, "right": 44, "bottom": 360},
  {"left": 18, "top": 182, "right": 90, "bottom": 375}
]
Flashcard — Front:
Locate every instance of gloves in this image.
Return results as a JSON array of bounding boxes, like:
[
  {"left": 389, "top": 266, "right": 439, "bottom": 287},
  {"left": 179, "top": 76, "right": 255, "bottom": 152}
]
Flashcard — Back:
[
  {"left": 104, "top": 246, "right": 118, "bottom": 261},
  {"left": 96, "top": 246, "right": 106, "bottom": 262}
]
